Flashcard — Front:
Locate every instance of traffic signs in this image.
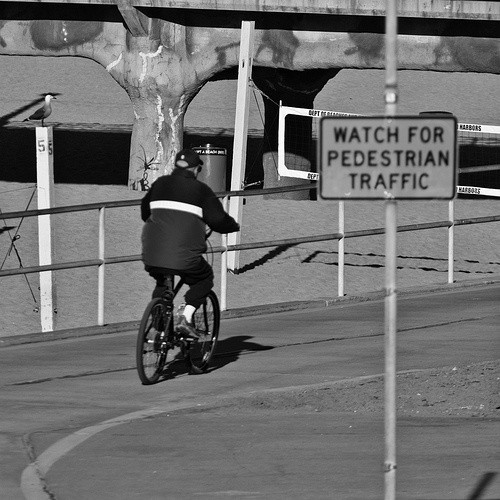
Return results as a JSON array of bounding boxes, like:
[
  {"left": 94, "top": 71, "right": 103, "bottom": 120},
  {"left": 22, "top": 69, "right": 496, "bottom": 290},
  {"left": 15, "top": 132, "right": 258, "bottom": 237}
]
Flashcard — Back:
[{"left": 315, "top": 112, "right": 459, "bottom": 203}]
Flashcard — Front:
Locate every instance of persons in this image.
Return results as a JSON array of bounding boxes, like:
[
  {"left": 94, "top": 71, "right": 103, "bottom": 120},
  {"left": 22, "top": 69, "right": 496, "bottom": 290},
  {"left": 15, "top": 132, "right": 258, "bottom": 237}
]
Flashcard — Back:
[{"left": 136, "top": 148, "right": 242, "bottom": 341}]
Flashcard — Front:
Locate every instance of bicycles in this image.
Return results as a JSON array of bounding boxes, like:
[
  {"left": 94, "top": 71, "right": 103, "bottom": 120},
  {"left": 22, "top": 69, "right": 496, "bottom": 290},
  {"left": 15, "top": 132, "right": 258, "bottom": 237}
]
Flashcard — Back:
[{"left": 135, "top": 228, "right": 221, "bottom": 385}]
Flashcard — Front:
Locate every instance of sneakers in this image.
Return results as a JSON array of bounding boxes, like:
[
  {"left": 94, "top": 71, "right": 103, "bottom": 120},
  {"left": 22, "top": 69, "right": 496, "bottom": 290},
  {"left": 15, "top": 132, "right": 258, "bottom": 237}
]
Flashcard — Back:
[
  {"left": 147, "top": 328, "right": 167, "bottom": 344},
  {"left": 176, "top": 315, "right": 201, "bottom": 338}
]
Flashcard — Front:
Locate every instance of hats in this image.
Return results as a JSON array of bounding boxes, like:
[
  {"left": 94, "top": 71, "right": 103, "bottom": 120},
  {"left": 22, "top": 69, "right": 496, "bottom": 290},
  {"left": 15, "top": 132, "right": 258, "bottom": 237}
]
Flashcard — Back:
[{"left": 175, "top": 149, "right": 203, "bottom": 168}]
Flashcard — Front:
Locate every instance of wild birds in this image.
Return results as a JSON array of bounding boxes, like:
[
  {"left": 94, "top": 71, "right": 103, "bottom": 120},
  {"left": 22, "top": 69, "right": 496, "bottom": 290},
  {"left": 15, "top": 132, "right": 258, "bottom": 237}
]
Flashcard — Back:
[{"left": 22, "top": 94, "right": 56, "bottom": 126}]
